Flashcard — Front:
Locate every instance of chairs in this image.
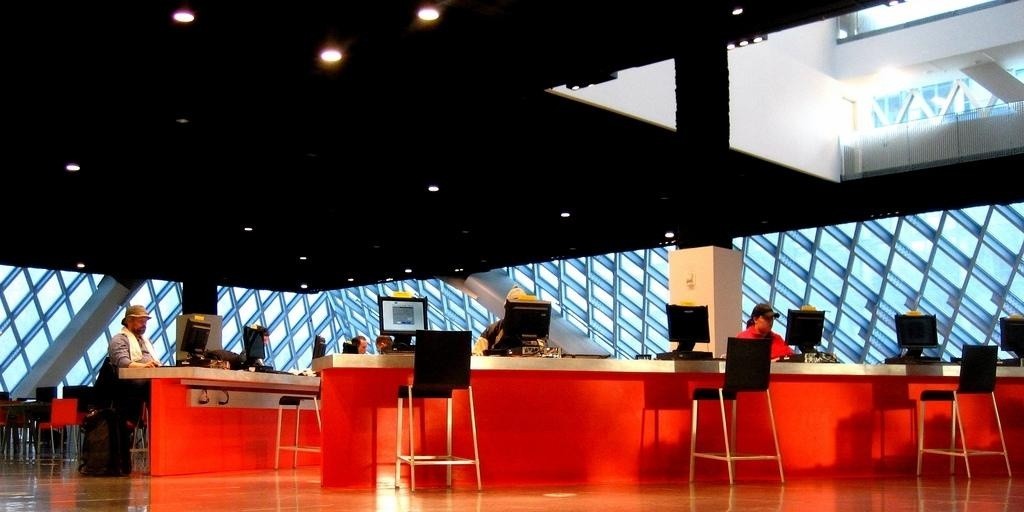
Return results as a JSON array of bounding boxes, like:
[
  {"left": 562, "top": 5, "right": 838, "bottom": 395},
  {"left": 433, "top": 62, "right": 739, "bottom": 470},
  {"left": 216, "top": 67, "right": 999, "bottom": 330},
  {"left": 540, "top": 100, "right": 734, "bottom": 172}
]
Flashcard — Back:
[
  {"left": 393, "top": 330, "right": 484, "bottom": 488},
  {"left": 690, "top": 335, "right": 786, "bottom": 490},
  {"left": 275, "top": 380, "right": 322, "bottom": 471},
  {"left": 0, "top": 384, "right": 91, "bottom": 474},
  {"left": 918, "top": 341, "right": 1010, "bottom": 483}
]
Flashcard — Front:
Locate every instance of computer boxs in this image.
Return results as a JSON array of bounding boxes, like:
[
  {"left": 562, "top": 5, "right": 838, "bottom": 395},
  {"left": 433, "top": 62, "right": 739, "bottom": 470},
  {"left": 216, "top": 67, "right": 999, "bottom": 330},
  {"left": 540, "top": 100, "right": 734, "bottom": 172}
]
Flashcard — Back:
[
  {"left": 657, "top": 352, "right": 713, "bottom": 361},
  {"left": 1001, "top": 359, "right": 1021, "bottom": 366},
  {"left": 780, "top": 355, "right": 820, "bottom": 362},
  {"left": 483, "top": 349, "right": 523, "bottom": 356},
  {"left": 238, "top": 365, "right": 273, "bottom": 372},
  {"left": 176, "top": 360, "right": 211, "bottom": 368},
  {"left": 885, "top": 357, "right": 940, "bottom": 365}
]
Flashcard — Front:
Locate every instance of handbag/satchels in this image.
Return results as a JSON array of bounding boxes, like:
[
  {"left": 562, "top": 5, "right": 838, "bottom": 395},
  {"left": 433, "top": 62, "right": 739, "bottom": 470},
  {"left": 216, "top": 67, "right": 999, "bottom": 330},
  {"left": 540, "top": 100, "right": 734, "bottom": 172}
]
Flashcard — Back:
[{"left": 78, "top": 410, "right": 132, "bottom": 476}]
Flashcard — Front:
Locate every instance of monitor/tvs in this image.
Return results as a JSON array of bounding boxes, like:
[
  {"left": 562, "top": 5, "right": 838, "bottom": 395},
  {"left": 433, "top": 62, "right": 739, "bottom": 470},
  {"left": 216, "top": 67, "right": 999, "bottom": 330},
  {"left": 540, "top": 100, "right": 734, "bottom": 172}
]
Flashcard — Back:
[
  {"left": 343, "top": 342, "right": 358, "bottom": 354},
  {"left": 313, "top": 335, "right": 327, "bottom": 359},
  {"left": 378, "top": 294, "right": 427, "bottom": 351},
  {"left": 666, "top": 304, "right": 710, "bottom": 352},
  {"left": 506, "top": 299, "right": 551, "bottom": 348},
  {"left": 244, "top": 326, "right": 265, "bottom": 366},
  {"left": 180, "top": 318, "right": 211, "bottom": 360},
  {"left": 785, "top": 309, "right": 825, "bottom": 355},
  {"left": 895, "top": 313, "right": 938, "bottom": 357},
  {"left": 1000, "top": 318, "right": 1024, "bottom": 359}
]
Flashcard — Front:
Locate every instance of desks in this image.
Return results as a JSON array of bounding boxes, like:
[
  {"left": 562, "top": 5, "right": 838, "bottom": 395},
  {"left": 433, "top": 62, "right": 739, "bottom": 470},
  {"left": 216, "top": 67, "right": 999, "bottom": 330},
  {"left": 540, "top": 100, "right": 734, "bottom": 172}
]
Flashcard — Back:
[
  {"left": 118, "top": 368, "right": 321, "bottom": 478},
  {"left": 315, "top": 350, "right": 1023, "bottom": 479}
]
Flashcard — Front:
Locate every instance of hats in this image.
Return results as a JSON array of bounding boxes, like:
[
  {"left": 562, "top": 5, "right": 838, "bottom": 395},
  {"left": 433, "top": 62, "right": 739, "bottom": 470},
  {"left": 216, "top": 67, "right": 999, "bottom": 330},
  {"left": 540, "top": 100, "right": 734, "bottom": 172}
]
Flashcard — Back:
[
  {"left": 503, "top": 285, "right": 526, "bottom": 308},
  {"left": 751, "top": 304, "right": 780, "bottom": 318},
  {"left": 120, "top": 305, "right": 152, "bottom": 318},
  {"left": 252, "top": 324, "right": 269, "bottom": 335}
]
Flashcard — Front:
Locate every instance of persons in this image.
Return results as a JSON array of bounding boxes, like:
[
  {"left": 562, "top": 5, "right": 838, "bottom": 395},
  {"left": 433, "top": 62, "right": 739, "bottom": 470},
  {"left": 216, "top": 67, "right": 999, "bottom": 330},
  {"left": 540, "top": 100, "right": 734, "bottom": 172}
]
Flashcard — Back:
[
  {"left": 256, "top": 325, "right": 272, "bottom": 369},
  {"left": 472, "top": 287, "right": 530, "bottom": 355},
  {"left": 736, "top": 303, "right": 797, "bottom": 358},
  {"left": 375, "top": 334, "right": 393, "bottom": 353},
  {"left": 109, "top": 305, "right": 162, "bottom": 417},
  {"left": 89, "top": 353, "right": 142, "bottom": 477},
  {"left": 351, "top": 336, "right": 369, "bottom": 354}
]
